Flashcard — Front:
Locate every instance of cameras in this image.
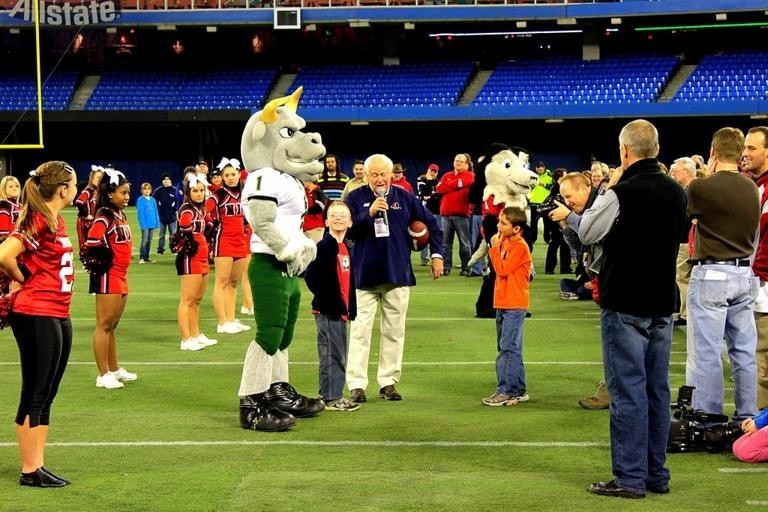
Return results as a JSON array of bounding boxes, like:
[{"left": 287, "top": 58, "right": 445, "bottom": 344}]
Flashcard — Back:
[{"left": 536, "top": 194, "right": 565, "bottom": 215}]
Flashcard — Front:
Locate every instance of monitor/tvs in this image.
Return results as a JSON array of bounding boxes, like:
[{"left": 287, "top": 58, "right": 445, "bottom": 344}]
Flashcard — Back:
[{"left": 274, "top": 8, "right": 301, "bottom": 30}]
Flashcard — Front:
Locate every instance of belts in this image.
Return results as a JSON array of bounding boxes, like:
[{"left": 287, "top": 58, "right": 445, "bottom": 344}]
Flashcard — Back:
[{"left": 693, "top": 255, "right": 750, "bottom": 267}]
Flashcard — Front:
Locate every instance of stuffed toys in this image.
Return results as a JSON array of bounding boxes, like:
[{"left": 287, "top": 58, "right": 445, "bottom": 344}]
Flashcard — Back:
[{"left": 238, "top": 87, "right": 324, "bottom": 430}]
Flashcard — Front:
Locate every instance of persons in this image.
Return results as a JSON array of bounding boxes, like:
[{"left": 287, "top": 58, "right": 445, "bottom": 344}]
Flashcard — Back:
[{"left": 1, "top": 117, "right": 768, "bottom": 499}]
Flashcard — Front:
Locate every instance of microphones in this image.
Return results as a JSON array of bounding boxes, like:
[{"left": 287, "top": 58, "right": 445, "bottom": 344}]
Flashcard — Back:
[{"left": 377, "top": 187, "right": 388, "bottom": 225}]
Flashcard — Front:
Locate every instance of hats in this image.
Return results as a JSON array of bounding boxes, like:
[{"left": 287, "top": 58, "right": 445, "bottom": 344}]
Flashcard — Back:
[
  {"left": 428, "top": 164, "right": 439, "bottom": 173},
  {"left": 392, "top": 164, "right": 404, "bottom": 173}
]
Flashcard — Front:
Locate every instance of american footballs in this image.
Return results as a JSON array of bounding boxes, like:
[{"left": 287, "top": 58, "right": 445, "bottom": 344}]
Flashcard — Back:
[{"left": 408, "top": 221, "right": 429, "bottom": 252}]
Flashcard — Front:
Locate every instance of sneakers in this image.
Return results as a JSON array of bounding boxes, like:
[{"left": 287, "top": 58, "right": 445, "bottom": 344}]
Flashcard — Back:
[{"left": 481, "top": 291, "right": 669, "bottom": 498}]
